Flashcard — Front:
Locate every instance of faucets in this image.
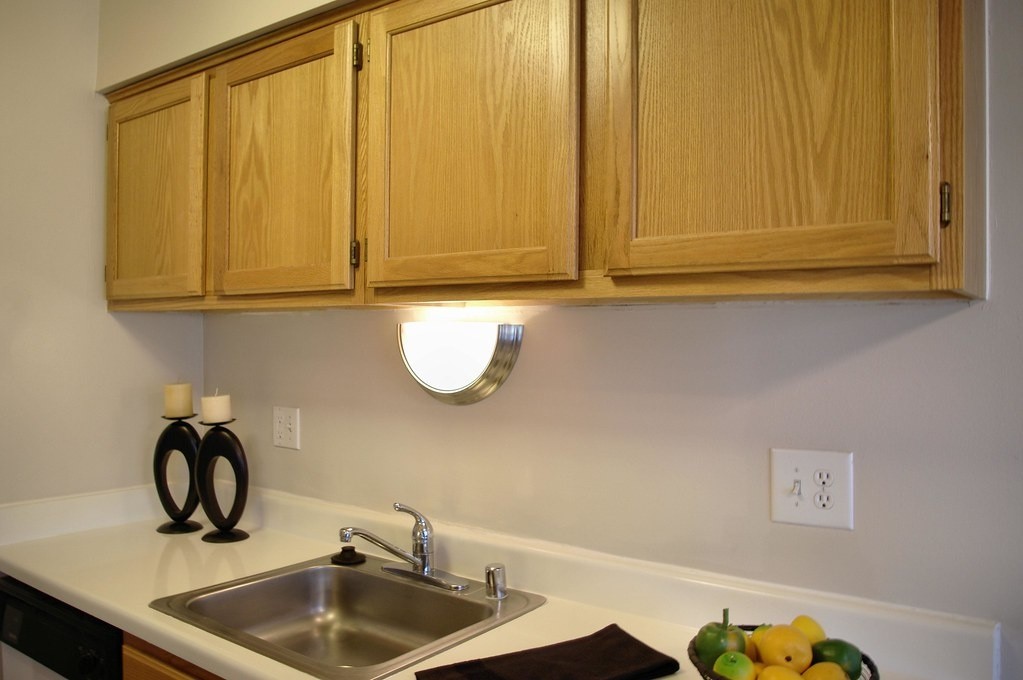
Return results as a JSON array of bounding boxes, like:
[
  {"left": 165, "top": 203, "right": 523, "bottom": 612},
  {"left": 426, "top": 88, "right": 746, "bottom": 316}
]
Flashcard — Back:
[{"left": 339, "top": 502, "right": 471, "bottom": 591}]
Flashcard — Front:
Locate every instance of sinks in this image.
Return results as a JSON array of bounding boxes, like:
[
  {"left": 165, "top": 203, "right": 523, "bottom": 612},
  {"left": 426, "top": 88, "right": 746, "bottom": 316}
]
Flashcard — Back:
[{"left": 147, "top": 550, "right": 547, "bottom": 680}]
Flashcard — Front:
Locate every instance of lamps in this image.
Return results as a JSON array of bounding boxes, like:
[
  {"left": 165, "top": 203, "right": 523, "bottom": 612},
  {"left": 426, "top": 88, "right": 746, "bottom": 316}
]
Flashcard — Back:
[{"left": 397, "top": 323, "right": 524, "bottom": 406}]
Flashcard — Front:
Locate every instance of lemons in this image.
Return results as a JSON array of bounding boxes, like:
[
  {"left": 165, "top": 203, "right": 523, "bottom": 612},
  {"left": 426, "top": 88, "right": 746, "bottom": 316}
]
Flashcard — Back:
[
  {"left": 809, "top": 639, "right": 862, "bottom": 680},
  {"left": 713, "top": 614, "right": 851, "bottom": 680}
]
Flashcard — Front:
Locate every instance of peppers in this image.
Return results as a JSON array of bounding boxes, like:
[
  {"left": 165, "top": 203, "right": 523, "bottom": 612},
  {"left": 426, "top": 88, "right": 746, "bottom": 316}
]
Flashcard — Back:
[{"left": 694, "top": 607, "right": 745, "bottom": 669}]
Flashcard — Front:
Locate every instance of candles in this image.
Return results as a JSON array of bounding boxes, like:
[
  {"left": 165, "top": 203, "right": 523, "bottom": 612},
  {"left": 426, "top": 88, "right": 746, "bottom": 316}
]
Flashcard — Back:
[
  {"left": 200, "top": 385, "right": 232, "bottom": 424},
  {"left": 161, "top": 377, "right": 192, "bottom": 417}
]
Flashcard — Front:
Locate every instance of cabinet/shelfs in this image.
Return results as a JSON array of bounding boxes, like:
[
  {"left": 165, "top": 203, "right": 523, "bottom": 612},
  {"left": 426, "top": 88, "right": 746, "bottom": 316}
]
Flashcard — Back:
[
  {"left": 366, "top": 0, "right": 969, "bottom": 308},
  {"left": 103, "top": 1, "right": 362, "bottom": 309}
]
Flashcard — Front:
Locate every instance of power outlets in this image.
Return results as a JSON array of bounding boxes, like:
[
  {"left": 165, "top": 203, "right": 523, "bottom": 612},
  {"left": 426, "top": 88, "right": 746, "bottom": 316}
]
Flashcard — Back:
[
  {"left": 771, "top": 449, "right": 853, "bottom": 529},
  {"left": 272, "top": 404, "right": 301, "bottom": 451}
]
